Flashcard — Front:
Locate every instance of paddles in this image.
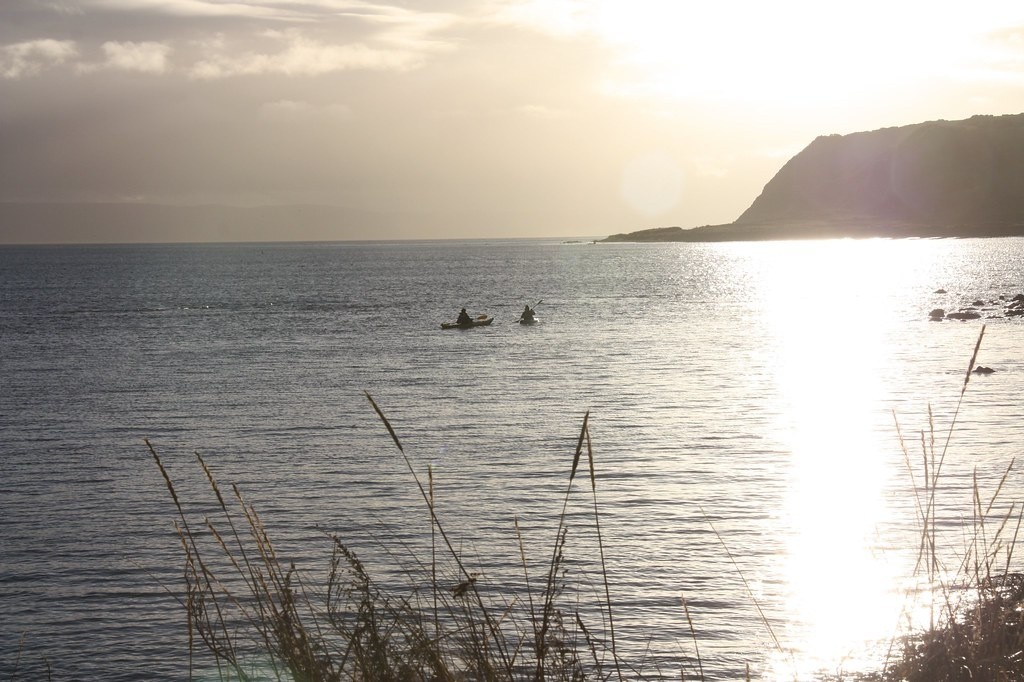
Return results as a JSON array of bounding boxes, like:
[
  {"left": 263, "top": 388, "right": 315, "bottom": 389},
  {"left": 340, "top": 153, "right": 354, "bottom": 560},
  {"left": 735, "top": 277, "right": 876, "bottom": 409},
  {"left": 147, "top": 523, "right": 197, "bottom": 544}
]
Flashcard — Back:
[
  {"left": 515, "top": 300, "right": 543, "bottom": 323},
  {"left": 450, "top": 315, "right": 488, "bottom": 325}
]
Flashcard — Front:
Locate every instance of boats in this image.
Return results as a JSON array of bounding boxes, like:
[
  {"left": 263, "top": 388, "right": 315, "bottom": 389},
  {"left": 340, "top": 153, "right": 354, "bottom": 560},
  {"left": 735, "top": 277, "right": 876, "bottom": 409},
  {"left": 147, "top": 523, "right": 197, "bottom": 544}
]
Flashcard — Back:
[
  {"left": 521, "top": 317, "right": 540, "bottom": 326},
  {"left": 440, "top": 317, "right": 494, "bottom": 330}
]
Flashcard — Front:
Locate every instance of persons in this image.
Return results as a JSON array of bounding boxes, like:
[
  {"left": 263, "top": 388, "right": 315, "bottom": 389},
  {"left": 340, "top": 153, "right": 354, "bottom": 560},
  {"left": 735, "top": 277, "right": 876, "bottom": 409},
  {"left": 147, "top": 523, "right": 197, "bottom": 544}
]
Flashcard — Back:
[
  {"left": 457, "top": 309, "right": 473, "bottom": 324},
  {"left": 521, "top": 305, "right": 535, "bottom": 321}
]
